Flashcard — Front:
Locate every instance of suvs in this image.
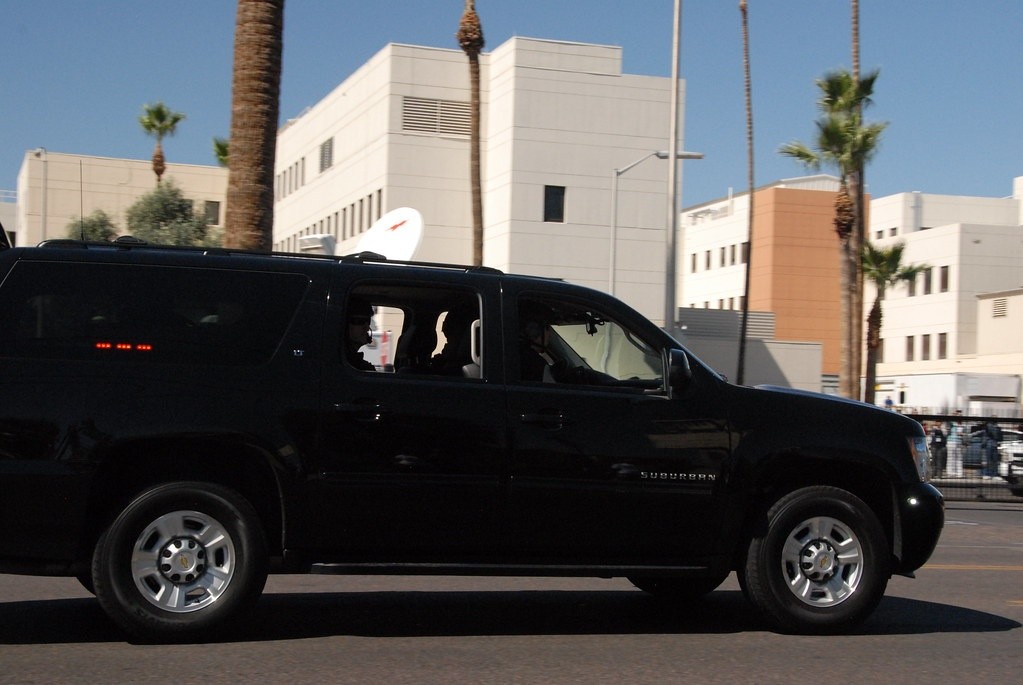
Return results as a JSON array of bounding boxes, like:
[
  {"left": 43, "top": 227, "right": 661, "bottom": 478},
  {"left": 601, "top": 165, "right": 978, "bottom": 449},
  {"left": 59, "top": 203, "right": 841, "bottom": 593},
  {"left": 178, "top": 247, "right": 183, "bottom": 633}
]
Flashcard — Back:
[{"left": 1, "top": 231, "right": 947, "bottom": 647}]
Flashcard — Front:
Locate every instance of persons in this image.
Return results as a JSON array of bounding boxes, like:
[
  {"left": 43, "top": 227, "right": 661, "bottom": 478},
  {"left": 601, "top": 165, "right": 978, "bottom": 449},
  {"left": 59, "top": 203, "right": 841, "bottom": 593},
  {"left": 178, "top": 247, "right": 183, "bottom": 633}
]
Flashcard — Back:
[
  {"left": 981, "top": 414, "right": 1003, "bottom": 480},
  {"left": 430, "top": 309, "right": 482, "bottom": 380},
  {"left": 333, "top": 300, "right": 381, "bottom": 371},
  {"left": 926, "top": 419, "right": 949, "bottom": 479},
  {"left": 941, "top": 409, "right": 967, "bottom": 478}
]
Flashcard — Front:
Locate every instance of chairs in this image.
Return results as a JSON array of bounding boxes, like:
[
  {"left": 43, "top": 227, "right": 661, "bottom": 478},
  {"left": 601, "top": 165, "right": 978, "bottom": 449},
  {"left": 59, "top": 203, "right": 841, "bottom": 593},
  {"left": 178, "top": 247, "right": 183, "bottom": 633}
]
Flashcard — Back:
[{"left": 394, "top": 322, "right": 438, "bottom": 378}]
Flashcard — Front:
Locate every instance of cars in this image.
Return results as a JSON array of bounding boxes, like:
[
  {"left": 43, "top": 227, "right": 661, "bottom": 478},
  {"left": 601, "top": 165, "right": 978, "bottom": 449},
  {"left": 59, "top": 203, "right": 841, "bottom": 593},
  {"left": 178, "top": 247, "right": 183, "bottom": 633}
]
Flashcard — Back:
[{"left": 966, "top": 428, "right": 1023, "bottom": 481}]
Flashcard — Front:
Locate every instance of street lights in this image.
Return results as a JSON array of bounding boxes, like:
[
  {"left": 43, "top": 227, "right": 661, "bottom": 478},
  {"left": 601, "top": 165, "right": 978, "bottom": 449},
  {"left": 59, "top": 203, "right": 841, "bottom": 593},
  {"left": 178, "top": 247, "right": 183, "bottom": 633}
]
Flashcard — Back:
[{"left": 602, "top": 149, "right": 705, "bottom": 376}]
